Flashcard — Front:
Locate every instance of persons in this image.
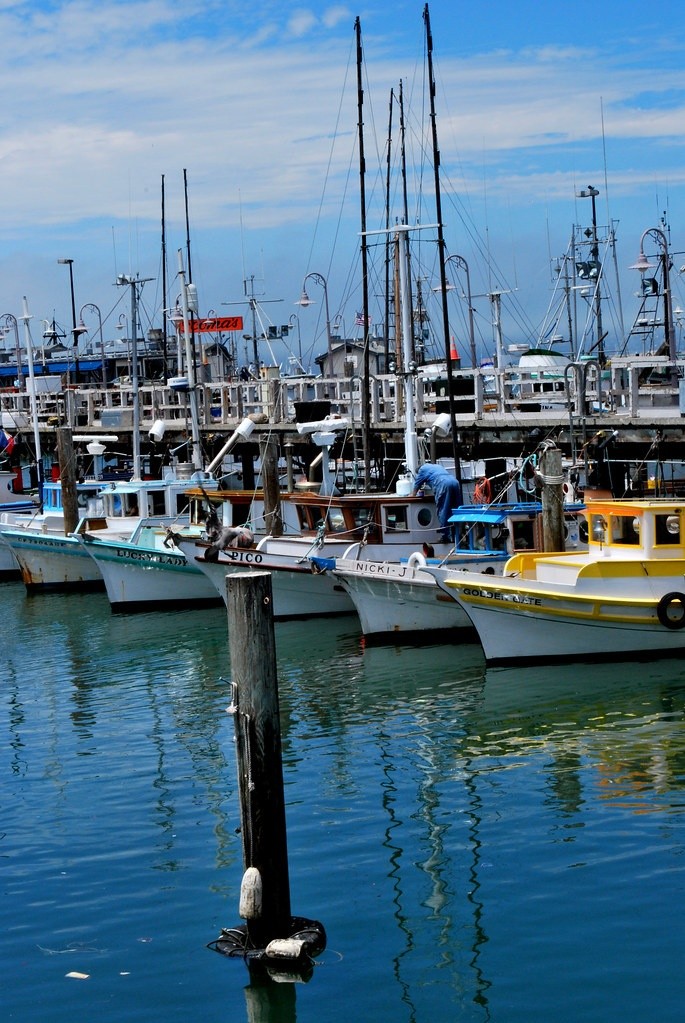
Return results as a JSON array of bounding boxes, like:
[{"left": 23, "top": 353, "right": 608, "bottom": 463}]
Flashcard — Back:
[{"left": 408, "top": 463, "right": 465, "bottom": 543}]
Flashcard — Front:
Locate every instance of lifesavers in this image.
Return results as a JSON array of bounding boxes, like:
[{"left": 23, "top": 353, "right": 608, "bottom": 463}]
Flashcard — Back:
[
  {"left": 407, "top": 551, "right": 427, "bottom": 568},
  {"left": 656, "top": 591, "right": 685, "bottom": 631}
]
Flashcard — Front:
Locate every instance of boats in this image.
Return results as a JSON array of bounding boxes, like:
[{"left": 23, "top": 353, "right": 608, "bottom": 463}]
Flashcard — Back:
[
  {"left": 0, "top": 1, "right": 685, "bottom": 649},
  {"left": 416, "top": 459, "right": 685, "bottom": 666}
]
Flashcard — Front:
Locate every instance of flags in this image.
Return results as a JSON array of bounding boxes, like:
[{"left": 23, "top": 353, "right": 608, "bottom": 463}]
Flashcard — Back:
[
  {"left": 356, "top": 313, "right": 370, "bottom": 327},
  {"left": 2, "top": 429, "right": 15, "bottom": 454}
]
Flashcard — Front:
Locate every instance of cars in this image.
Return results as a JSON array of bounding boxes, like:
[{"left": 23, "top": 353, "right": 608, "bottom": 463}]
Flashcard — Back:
[{"left": 110, "top": 373, "right": 152, "bottom": 386}]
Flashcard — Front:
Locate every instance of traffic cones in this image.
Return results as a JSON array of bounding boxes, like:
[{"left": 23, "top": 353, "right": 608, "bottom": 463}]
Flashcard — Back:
[
  {"left": 202, "top": 344, "right": 208, "bottom": 365},
  {"left": 450, "top": 335, "right": 462, "bottom": 360}
]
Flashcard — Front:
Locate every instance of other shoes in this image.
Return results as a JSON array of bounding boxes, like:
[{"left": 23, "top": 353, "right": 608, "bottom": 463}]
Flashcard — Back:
[{"left": 440, "top": 537, "right": 451, "bottom": 544}]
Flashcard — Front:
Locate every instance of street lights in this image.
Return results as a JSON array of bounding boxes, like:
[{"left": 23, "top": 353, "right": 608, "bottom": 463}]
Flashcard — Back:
[
  {"left": 58, "top": 258, "right": 80, "bottom": 382},
  {"left": 70, "top": 302, "right": 109, "bottom": 391},
  {"left": 431, "top": 253, "right": 478, "bottom": 369},
  {"left": 0, "top": 312, "right": 25, "bottom": 394},
  {"left": 293, "top": 273, "right": 338, "bottom": 415},
  {"left": 333, "top": 315, "right": 348, "bottom": 364},
  {"left": 202, "top": 308, "right": 222, "bottom": 383},
  {"left": 287, "top": 314, "right": 304, "bottom": 375},
  {"left": 114, "top": 313, "right": 132, "bottom": 384},
  {"left": 627, "top": 227, "right": 680, "bottom": 405},
  {"left": 41, "top": 319, "right": 56, "bottom": 375}
]
